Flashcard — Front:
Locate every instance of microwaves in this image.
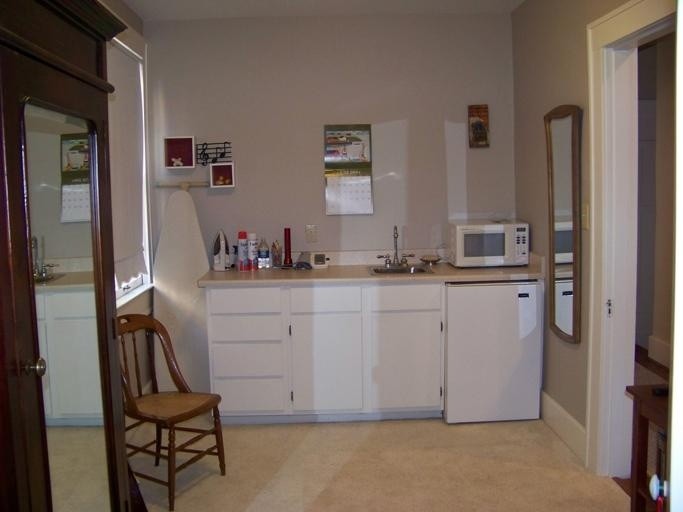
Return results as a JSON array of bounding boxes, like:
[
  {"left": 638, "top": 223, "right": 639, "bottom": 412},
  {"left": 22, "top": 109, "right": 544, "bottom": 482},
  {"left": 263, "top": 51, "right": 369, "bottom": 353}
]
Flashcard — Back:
[
  {"left": 447, "top": 218, "right": 530, "bottom": 268},
  {"left": 553, "top": 221, "right": 574, "bottom": 264}
]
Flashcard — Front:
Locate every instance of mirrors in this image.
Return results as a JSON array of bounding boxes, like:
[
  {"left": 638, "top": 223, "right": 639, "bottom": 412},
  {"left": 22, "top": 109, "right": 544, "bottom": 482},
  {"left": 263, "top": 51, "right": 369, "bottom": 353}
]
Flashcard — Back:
[{"left": 20, "top": 93, "right": 119, "bottom": 512}]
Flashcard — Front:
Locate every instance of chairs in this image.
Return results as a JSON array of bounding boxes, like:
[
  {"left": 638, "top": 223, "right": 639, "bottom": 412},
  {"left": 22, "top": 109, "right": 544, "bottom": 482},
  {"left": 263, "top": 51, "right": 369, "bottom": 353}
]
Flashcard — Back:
[{"left": 111, "top": 311, "right": 228, "bottom": 512}]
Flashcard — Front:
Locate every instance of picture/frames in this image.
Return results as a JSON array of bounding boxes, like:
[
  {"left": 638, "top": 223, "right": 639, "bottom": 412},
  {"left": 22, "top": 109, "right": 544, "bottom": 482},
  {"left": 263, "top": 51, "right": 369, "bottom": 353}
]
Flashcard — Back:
[
  {"left": 208, "top": 162, "right": 235, "bottom": 190},
  {"left": 162, "top": 135, "right": 195, "bottom": 170}
]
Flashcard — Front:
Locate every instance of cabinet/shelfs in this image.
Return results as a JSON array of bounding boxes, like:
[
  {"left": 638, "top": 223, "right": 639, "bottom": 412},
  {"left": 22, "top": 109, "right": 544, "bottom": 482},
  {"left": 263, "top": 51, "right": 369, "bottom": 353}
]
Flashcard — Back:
[
  {"left": 0, "top": 0, "right": 142, "bottom": 512},
  {"left": 289, "top": 283, "right": 444, "bottom": 414},
  {"left": 621, "top": 380, "right": 669, "bottom": 512},
  {"left": 542, "top": 106, "right": 584, "bottom": 345},
  {"left": 204, "top": 286, "right": 288, "bottom": 418}
]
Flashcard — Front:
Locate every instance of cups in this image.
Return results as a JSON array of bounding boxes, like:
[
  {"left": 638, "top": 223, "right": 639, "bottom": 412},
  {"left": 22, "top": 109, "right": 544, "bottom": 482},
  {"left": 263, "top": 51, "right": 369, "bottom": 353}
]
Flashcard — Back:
[{"left": 271, "top": 247, "right": 283, "bottom": 266}]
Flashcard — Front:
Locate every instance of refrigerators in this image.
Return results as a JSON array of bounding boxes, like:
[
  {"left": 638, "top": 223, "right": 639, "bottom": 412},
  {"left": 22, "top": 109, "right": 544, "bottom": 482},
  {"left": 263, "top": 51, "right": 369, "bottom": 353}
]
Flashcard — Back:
[
  {"left": 553, "top": 279, "right": 573, "bottom": 337},
  {"left": 443, "top": 280, "right": 546, "bottom": 424}
]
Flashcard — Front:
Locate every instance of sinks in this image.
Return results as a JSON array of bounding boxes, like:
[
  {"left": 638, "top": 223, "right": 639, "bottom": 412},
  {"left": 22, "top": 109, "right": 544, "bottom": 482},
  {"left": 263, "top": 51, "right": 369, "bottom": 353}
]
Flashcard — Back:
[{"left": 370, "top": 265, "right": 435, "bottom": 275}]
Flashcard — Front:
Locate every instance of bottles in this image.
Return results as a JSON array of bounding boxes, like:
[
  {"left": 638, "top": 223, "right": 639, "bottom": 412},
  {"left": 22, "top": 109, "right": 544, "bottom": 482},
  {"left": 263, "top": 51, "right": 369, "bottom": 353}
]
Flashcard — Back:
[{"left": 236, "top": 230, "right": 270, "bottom": 272}]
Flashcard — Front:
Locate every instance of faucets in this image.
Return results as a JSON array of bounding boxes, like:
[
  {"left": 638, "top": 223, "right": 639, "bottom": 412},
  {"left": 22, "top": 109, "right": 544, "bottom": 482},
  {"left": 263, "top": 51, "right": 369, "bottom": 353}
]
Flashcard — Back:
[
  {"left": 31, "top": 235, "right": 38, "bottom": 269},
  {"left": 394, "top": 225, "right": 398, "bottom": 265}
]
